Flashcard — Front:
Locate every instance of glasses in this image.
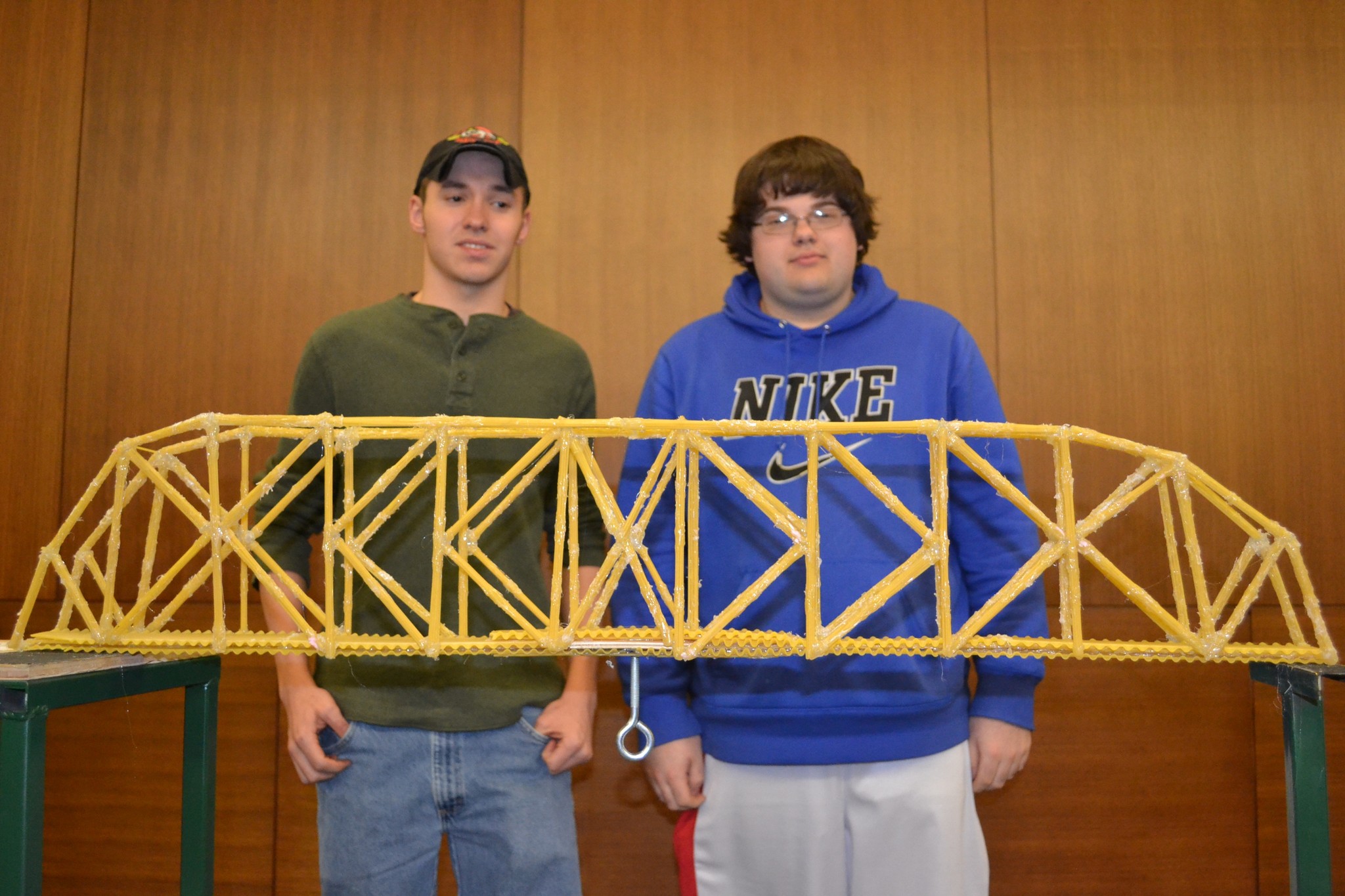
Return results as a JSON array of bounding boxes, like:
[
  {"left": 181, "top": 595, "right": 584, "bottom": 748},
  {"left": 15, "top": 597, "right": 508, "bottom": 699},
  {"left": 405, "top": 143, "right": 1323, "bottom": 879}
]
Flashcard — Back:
[{"left": 751, "top": 206, "right": 849, "bottom": 234}]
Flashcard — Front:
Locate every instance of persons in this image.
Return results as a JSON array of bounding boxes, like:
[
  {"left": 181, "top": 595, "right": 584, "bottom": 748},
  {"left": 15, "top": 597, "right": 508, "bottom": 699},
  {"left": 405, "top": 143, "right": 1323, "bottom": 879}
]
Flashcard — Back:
[
  {"left": 612, "top": 135, "right": 1050, "bottom": 896},
  {"left": 254, "top": 126, "right": 613, "bottom": 896}
]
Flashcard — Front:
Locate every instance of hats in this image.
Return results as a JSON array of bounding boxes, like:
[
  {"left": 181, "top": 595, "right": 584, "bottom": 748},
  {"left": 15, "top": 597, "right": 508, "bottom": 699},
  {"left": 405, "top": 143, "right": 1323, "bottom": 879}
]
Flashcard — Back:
[{"left": 415, "top": 125, "right": 530, "bottom": 210}]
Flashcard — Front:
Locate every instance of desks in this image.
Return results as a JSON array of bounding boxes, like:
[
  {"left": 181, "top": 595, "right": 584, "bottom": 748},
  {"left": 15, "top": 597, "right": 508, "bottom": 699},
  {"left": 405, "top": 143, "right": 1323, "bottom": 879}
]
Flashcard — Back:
[{"left": 0, "top": 637, "right": 222, "bottom": 895}]
